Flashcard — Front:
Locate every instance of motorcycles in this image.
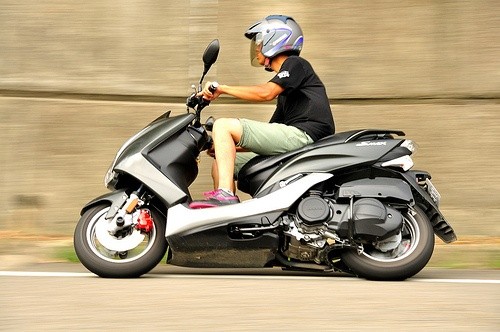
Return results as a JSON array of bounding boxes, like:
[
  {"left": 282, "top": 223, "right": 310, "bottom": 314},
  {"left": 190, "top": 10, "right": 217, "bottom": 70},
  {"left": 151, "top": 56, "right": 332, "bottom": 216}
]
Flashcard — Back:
[{"left": 72, "top": 39, "right": 456, "bottom": 281}]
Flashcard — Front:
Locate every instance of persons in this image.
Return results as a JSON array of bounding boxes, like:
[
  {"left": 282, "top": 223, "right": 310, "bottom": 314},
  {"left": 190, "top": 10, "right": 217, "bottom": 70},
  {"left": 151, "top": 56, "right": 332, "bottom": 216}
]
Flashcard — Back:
[{"left": 188, "top": 14, "right": 335, "bottom": 209}]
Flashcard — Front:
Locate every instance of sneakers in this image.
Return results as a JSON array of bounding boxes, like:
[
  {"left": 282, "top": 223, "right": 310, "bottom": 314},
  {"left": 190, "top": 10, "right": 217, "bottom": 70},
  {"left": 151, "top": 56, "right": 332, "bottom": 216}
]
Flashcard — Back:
[{"left": 189, "top": 189, "right": 240, "bottom": 209}]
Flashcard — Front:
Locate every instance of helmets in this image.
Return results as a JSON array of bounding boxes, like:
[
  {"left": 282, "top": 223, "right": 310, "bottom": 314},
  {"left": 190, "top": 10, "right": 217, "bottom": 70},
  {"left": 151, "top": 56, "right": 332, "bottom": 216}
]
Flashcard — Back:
[{"left": 245, "top": 14, "right": 303, "bottom": 59}]
